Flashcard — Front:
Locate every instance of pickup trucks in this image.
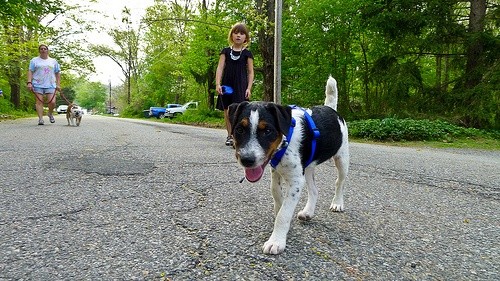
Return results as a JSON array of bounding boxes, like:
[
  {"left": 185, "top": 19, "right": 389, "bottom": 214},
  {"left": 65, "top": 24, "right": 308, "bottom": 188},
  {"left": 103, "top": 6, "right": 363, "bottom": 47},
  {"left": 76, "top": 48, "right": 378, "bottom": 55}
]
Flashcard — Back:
[
  {"left": 149, "top": 103, "right": 183, "bottom": 119},
  {"left": 164, "top": 102, "right": 220, "bottom": 120}
]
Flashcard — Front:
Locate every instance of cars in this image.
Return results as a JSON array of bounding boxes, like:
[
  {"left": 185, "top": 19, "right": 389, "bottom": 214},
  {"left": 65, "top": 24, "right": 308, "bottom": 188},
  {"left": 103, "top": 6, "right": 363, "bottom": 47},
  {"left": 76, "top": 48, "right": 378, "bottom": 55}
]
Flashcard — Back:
[{"left": 57, "top": 105, "right": 68, "bottom": 114}]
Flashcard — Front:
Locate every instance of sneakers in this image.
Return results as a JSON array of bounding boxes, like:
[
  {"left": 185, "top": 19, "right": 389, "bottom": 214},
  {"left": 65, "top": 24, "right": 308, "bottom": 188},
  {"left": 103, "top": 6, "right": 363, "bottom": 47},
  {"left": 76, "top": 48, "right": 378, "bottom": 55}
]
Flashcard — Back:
[{"left": 225, "top": 135, "right": 234, "bottom": 146}]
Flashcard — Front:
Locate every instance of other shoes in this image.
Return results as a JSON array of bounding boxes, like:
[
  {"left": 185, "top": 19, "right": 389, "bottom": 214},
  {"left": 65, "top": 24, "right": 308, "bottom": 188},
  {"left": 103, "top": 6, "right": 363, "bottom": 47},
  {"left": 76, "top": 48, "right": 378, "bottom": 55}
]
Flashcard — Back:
[
  {"left": 38, "top": 119, "right": 44, "bottom": 125},
  {"left": 50, "top": 116, "right": 55, "bottom": 123}
]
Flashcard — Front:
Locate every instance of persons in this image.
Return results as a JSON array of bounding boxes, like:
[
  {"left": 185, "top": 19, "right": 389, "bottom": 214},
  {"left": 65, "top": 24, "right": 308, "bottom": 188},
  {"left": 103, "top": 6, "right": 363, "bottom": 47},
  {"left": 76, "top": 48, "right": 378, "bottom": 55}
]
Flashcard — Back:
[
  {"left": 216, "top": 23, "right": 253, "bottom": 146},
  {"left": 28, "top": 45, "right": 61, "bottom": 125}
]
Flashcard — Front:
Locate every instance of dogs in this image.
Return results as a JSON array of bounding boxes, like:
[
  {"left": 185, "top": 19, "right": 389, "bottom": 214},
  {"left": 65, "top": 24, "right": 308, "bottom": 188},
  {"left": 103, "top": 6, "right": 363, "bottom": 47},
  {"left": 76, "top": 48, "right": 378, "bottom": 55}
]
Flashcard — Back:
[
  {"left": 66, "top": 104, "right": 84, "bottom": 127},
  {"left": 227, "top": 75, "right": 350, "bottom": 255}
]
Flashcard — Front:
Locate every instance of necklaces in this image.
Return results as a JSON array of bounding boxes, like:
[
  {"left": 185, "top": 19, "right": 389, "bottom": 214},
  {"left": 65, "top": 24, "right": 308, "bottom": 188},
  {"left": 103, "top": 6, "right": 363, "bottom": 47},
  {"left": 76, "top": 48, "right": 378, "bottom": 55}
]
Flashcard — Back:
[{"left": 230, "top": 45, "right": 243, "bottom": 60}]
